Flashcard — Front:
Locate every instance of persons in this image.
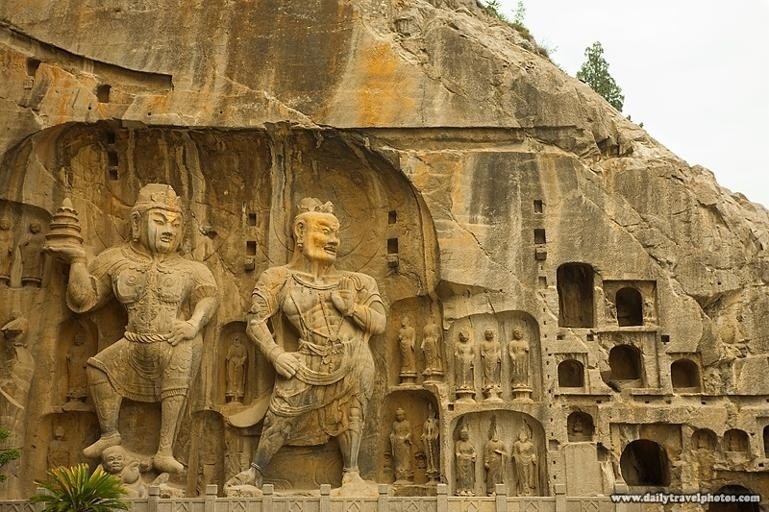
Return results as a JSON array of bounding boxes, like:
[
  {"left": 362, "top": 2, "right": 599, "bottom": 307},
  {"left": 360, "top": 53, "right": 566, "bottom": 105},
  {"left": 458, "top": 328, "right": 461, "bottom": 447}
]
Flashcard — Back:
[
  {"left": 19, "top": 221, "right": 46, "bottom": 283},
  {"left": 398, "top": 316, "right": 531, "bottom": 390},
  {"left": 0, "top": 217, "right": 15, "bottom": 278},
  {"left": 44, "top": 184, "right": 218, "bottom": 472},
  {"left": 390, "top": 403, "right": 538, "bottom": 498},
  {"left": 560, "top": 279, "right": 583, "bottom": 328},
  {"left": 226, "top": 336, "right": 248, "bottom": 399},
  {"left": 47, "top": 425, "right": 72, "bottom": 469},
  {"left": 573, "top": 416, "right": 582, "bottom": 427},
  {"left": 729, "top": 436, "right": 738, "bottom": 451},
  {"left": 64, "top": 332, "right": 92, "bottom": 398},
  {"left": 103, "top": 446, "right": 140, "bottom": 500},
  {"left": 697, "top": 436, "right": 704, "bottom": 449},
  {"left": 225, "top": 198, "right": 387, "bottom": 486}
]
